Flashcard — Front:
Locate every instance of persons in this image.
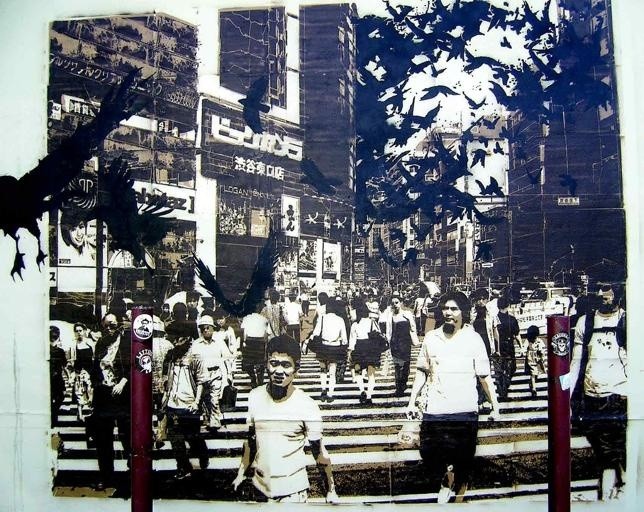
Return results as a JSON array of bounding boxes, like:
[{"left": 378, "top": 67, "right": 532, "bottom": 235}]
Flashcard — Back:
[
  {"left": 159, "top": 322, "right": 210, "bottom": 481},
  {"left": 385, "top": 295, "right": 416, "bottom": 396},
  {"left": 282, "top": 292, "right": 305, "bottom": 344},
  {"left": 568, "top": 275, "right": 627, "bottom": 502},
  {"left": 192, "top": 315, "right": 233, "bottom": 431},
  {"left": 67, "top": 323, "right": 96, "bottom": 421},
  {"left": 160, "top": 303, "right": 173, "bottom": 326},
  {"left": 468, "top": 287, "right": 501, "bottom": 410},
  {"left": 240, "top": 304, "right": 272, "bottom": 389},
  {"left": 378, "top": 292, "right": 388, "bottom": 312},
  {"left": 166, "top": 301, "right": 194, "bottom": 338},
  {"left": 300, "top": 290, "right": 309, "bottom": 316},
  {"left": 152, "top": 313, "right": 174, "bottom": 448},
  {"left": 185, "top": 291, "right": 202, "bottom": 322},
  {"left": 139, "top": 318, "right": 149, "bottom": 331},
  {"left": 139, "top": 355, "right": 152, "bottom": 374},
  {"left": 307, "top": 297, "right": 348, "bottom": 403},
  {"left": 518, "top": 326, "right": 546, "bottom": 398},
  {"left": 365, "top": 294, "right": 379, "bottom": 312},
  {"left": 90, "top": 313, "right": 131, "bottom": 491},
  {"left": 232, "top": 334, "right": 340, "bottom": 504},
  {"left": 215, "top": 309, "right": 238, "bottom": 370},
  {"left": 335, "top": 295, "right": 351, "bottom": 384},
  {"left": 312, "top": 292, "right": 328, "bottom": 327},
  {"left": 49, "top": 325, "right": 69, "bottom": 429},
  {"left": 347, "top": 301, "right": 382, "bottom": 406},
  {"left": 260, "top": 290, "right": 287, "bottom": 338},
  {"left": 496, "top": 297, "right": 523, "bottom": 399},
  {"left": 580, "top": 271, "right": 589, "bottom": 296},
  {"left": 405, "top": 293, "right": 500, "bottom": 505},
  {"left": 413, "top": 291, "right": 428, "bottom": 337}
]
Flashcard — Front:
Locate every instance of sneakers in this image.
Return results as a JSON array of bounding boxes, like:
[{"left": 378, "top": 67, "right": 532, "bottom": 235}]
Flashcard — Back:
[
  {"left": 173, "top": 470, "right": 193, "bottom": 480},
  {"left": 366, "top": 399, "right": 372, "bottom": 405},
  {"left": 321, "top": 390, "right": 327, "bottom": 402},
  {"left": 95, "top": 477, "right": 114, "bottom": 489},
  {"left": 360, "top": 391, "right": 367, "bottom": 405},
  {"left": 327, "top": 397, "right": 334, "bottom": 402},
  {"left": 437, "top": 470, "right": 455, "bottom": 504}
]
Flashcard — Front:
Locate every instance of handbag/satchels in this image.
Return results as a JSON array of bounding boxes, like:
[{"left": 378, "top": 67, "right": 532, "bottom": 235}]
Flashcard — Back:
[
  {"left": 219, "top": 379, "right": 238, "bottom": 412},
  {"left": 570, "top": 309, "right": 595, "bottom": 437},
  {"left": 235, "top": 426, "right": 268, "bottom": 503},
  {"left": 306, "top": 316, "right": 323, "bottom": 353},
  {"left": 242, "top": 349, "right": 254, "bottom": 372},
  {"left": 368, "top": 320, "right": 389, "bottom": 352}
]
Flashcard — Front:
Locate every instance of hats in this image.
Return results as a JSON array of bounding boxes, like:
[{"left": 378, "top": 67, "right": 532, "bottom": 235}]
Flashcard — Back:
[{"left": 198, "top": 315, "right": 216, "bottom": 326}]
